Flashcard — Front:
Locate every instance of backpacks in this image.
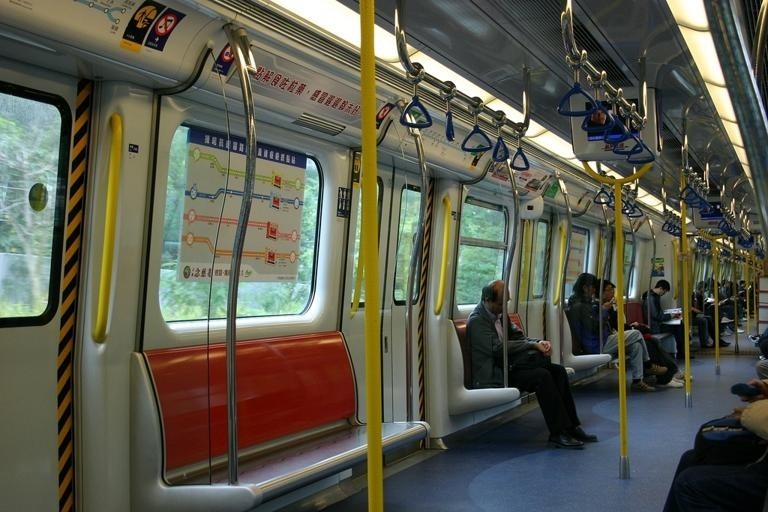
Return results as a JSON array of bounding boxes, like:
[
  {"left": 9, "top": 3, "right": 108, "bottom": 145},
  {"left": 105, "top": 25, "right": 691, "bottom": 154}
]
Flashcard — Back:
[{"left": 694, "top": 410, "right": 766, "bottom": 463}]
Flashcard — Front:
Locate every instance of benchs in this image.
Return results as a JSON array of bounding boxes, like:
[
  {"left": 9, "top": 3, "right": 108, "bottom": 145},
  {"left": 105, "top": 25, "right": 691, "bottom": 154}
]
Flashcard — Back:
[
  {"left": 442, "top": 300, "right": 669, "bottom": 418},
  {"left": 136, "top": 332, "right": 432, "bottom": 510}
]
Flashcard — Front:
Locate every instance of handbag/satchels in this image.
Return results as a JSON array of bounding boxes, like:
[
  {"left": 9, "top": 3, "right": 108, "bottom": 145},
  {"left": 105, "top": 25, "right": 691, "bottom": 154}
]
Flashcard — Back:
[{"left": 514, "top": 347, "right": 553, "bottom": 371}]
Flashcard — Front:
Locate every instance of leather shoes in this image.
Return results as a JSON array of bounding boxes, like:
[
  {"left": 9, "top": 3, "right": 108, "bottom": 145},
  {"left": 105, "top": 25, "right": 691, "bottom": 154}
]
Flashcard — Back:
[
  {"left": 548, "top": 430, "right": 586, "bottom": 450},
  {"left": 569, "top": 426, "right": 598, "bottom": 443}
]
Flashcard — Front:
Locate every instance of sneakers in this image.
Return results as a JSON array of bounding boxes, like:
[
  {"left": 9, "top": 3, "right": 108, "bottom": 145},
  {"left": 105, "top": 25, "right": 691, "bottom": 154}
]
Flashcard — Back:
[
  {"left": 643, "top": 362, "right": 669, "bottom": 376},
  {"left": 630, "top": 380, "right": 656, "bottom": 391},
  {"left": 748, "top": 334, "right": 762, "bottom": 344},
  {"left": 656, "top": 372, "right": 694, "bottom": 388},
  {"left": 719, "top": 328, "right": 745, "bottom": 348},
  {"left": 720, "top": 316, "right": 734, "bottom": 324}
]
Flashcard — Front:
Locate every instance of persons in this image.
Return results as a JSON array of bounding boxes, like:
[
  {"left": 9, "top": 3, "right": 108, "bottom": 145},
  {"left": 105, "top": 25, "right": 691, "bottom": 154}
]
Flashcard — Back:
[
  {"left": 749, "top": 324, "right": 768, "bottom": 360},
  {"left": 595, "top": 276, "right": 686, "bottom": 388},
  {"left": 640, "top": 279, "right": 695, "bottom": 361},
  {"left": 660, "top": 378, "right": 768, "bottom": 512},
  {"left": 755, "top": 359, "right": 768, "bottom": 380},
  {"left": 463, "top": 280, "right": 598, "bottom": 450},
  {"left": 691, "top": 271, "right": 758, "bottom": 348},
  {"left": 568, "top": 272, "right": 666, "bottom": 393}
]
